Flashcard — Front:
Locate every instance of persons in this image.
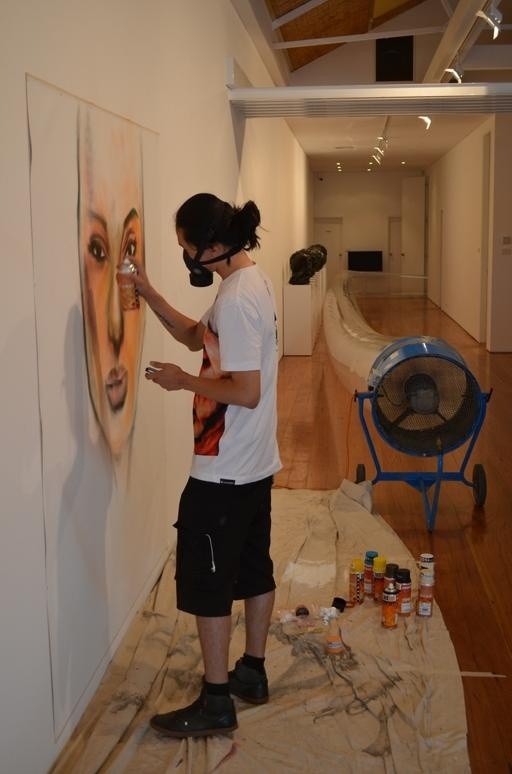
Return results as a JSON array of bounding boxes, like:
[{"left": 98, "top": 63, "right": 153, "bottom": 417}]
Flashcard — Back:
[
  {"left": 76, "top": 100, "right": 149, "bottom": 458},
  {"left": 123, "top": 192, "right": 282, "bottom": 737}
]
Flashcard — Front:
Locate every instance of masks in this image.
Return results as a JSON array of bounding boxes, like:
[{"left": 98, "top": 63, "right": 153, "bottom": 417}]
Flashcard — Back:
[{"left": 183, "top": 246, "right": 241, "bottom": 286}]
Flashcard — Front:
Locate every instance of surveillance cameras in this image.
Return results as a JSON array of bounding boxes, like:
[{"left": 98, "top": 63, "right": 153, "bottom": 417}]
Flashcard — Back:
[{"left": 318, "top": 176, "right": 326, "bottom": 184}]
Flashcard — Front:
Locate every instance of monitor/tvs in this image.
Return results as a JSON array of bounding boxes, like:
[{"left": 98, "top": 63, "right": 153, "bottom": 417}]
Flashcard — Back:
[{"left": 346, "top": 249, "right": 384, "bottom": 273}]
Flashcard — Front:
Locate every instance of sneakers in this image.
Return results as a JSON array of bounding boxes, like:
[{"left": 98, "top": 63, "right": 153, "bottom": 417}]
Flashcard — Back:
[
  {"left": 202, "top": 659, "right": 268, "bottom": 704},
  {"left": 150, "top": 693, "right": 238, "bottom": 737}
]
